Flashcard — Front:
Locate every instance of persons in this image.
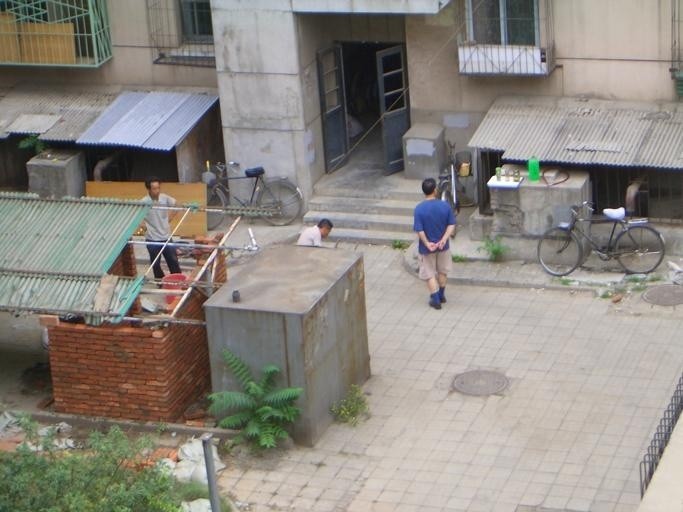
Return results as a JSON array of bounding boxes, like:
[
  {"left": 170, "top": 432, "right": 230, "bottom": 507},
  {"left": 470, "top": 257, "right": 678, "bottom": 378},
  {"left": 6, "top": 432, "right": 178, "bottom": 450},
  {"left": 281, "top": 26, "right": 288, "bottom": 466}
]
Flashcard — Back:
[
  {"left": 297, "top": 218, "right": 333, "bottom": 247},
  {"left": 139, "top": 175, "right": 184, "bottom": 287},
  {"left": 413, "top": 178, "right": 457, "bottom": 310}
]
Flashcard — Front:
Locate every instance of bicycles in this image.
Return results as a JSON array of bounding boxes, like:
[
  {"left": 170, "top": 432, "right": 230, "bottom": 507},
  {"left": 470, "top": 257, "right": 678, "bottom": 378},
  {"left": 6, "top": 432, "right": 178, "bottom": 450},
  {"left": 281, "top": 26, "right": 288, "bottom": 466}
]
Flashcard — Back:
[
  {"left": 535, "top": 201, "right": 670, "bottom": 276},
  {"left": 199, "top": 160, "right": 303, "bottom": 230},
  {"left": 434, "top": 139, "right": 473, "bottom": 215}
]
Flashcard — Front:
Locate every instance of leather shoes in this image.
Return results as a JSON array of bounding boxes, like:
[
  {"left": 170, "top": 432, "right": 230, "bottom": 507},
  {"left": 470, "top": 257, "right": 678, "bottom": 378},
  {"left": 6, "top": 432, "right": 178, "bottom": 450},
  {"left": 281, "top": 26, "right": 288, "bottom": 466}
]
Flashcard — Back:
[
  {"left": 429, "top": 301, "right": 442, "bottom": 309},
  {"left": 430, "top": 293, "right": 447, "bottom": 303}
]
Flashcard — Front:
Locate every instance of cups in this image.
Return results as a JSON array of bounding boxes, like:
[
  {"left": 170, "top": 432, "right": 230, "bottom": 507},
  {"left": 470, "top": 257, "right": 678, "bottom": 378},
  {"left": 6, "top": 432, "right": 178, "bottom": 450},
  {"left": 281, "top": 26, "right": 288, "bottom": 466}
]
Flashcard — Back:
[{"left": 496, "top": 167, "right": 520, "bottom": 182}]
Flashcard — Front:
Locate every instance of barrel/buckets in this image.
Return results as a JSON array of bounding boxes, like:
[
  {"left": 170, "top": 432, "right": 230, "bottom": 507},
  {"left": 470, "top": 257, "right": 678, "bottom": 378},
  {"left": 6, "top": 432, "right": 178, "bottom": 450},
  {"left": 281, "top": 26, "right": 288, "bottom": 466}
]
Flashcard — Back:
[{"left": 160, "top": 272, "right": 186, "bottom": 305}]
